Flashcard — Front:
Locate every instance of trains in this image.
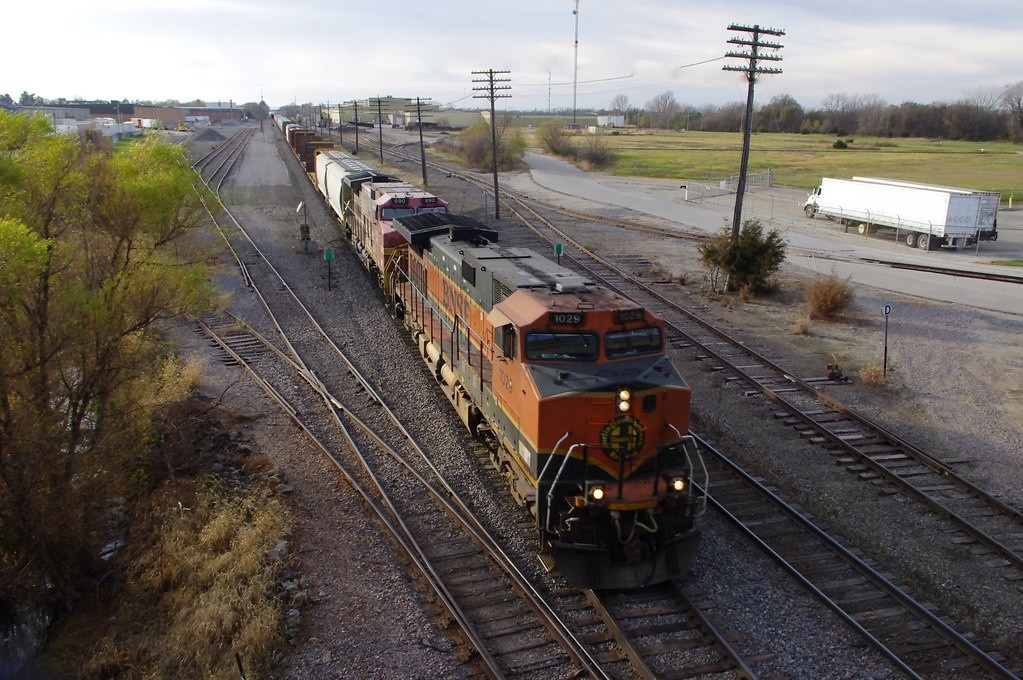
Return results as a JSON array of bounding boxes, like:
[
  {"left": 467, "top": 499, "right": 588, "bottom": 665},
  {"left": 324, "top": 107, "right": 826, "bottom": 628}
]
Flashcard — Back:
[{"left": 271, "top": 112, "right": 710, "bottom": 590}]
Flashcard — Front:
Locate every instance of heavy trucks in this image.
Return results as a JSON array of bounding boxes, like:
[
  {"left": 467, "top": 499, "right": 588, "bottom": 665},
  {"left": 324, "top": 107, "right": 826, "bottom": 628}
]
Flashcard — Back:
[{"left": 803, "top": 175, "right": 1001, "bottom": 252}]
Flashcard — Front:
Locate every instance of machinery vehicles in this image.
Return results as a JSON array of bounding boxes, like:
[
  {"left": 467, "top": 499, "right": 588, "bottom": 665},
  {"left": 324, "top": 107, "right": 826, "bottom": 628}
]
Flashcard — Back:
[{"left": 174, "top": 121, "right": 189, "bottom": 131}]
